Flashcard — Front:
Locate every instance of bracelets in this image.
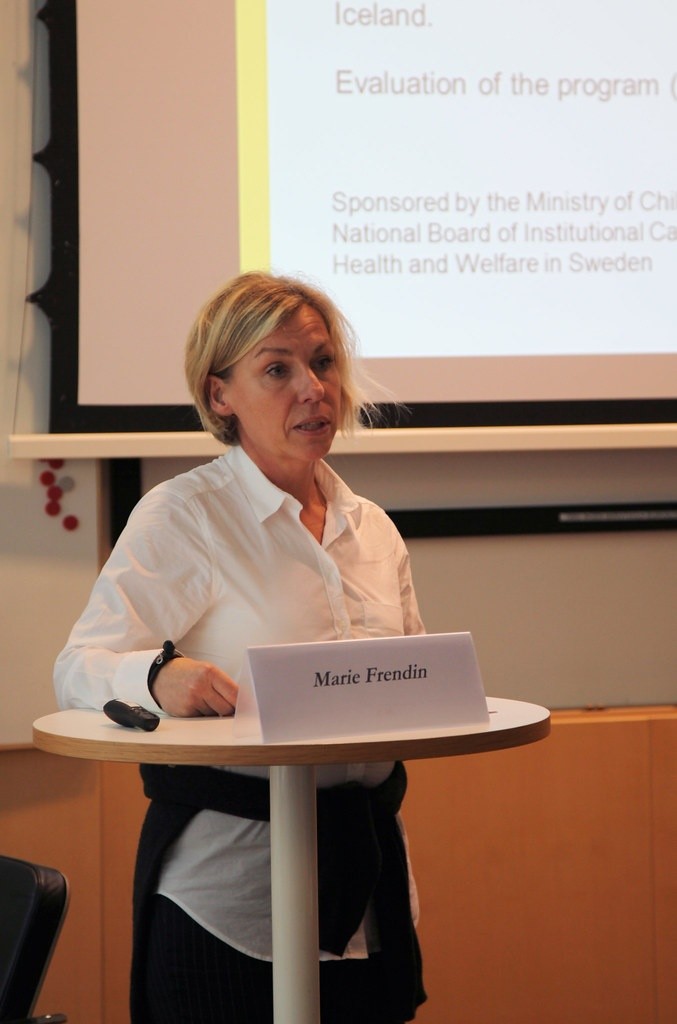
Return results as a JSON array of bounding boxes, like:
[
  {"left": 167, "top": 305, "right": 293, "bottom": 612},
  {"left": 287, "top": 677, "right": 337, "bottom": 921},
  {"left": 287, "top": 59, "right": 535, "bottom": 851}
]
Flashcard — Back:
[{"left": 147, "top": 640, "right": 184, "bottom": 691}]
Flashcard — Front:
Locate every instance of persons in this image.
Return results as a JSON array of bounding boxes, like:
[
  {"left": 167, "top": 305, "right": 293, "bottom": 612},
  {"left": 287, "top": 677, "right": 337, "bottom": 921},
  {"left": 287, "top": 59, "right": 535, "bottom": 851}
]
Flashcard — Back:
[{"left": 53, "top": 273, "right": 428, "bottom": 1024}]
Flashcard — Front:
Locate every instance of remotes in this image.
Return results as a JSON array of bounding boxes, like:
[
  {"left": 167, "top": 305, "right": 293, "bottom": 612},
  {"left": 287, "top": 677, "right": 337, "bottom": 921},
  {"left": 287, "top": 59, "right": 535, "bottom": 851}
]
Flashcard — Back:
[{"left": 103, "top": 697, "right": 160, "bottom": 731}]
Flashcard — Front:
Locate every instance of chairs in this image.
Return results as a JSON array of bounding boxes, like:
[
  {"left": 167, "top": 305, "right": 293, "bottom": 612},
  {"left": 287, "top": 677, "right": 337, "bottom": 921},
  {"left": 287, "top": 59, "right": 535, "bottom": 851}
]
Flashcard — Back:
[{"left": 0, "top": 855, "right": 71, "bottom": 1024}]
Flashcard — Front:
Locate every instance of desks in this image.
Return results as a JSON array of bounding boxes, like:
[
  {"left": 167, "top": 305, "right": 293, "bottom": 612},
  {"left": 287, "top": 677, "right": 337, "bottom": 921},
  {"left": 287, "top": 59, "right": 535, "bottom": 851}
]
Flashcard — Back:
[{"left": 32, "top": 696, "right": 552, "bottom": 1024}]
